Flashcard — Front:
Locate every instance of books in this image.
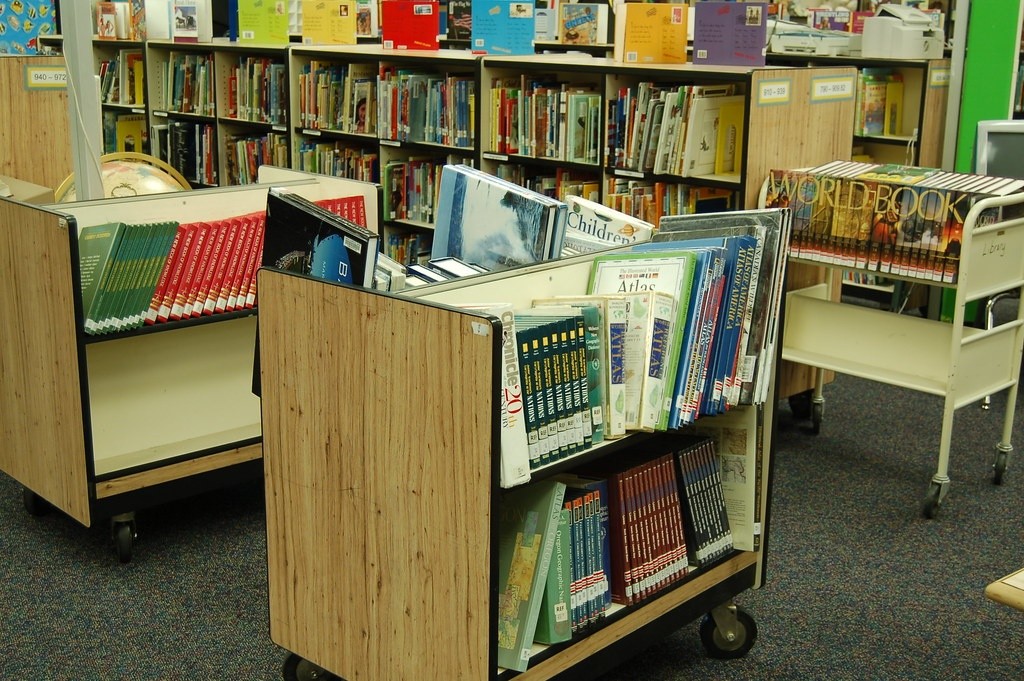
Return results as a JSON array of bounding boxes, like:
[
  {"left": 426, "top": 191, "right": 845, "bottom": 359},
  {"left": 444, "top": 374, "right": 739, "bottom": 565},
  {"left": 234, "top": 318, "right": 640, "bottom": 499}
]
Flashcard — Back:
[
  {"left": 79, "top": 196, "right": 367, "bottom": 334},
  {"left": 89, "top": 0, "right": 904, "bottom": 235},
  {"left": 499, "top": 405, "right": 764, "bottom": 673},
  {"left": 765, "top": 160, "right": 1024, "bottom": 282},
  {"left": 252, "top": 165, "right": 793, "bottom": 489}
]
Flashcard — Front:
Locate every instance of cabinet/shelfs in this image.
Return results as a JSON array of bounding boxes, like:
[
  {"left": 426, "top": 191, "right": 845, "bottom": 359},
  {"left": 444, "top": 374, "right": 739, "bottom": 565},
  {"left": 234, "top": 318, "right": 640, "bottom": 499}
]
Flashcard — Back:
[{"left": 0, "top": 0, "right": 1024, "bottom": 681}]
